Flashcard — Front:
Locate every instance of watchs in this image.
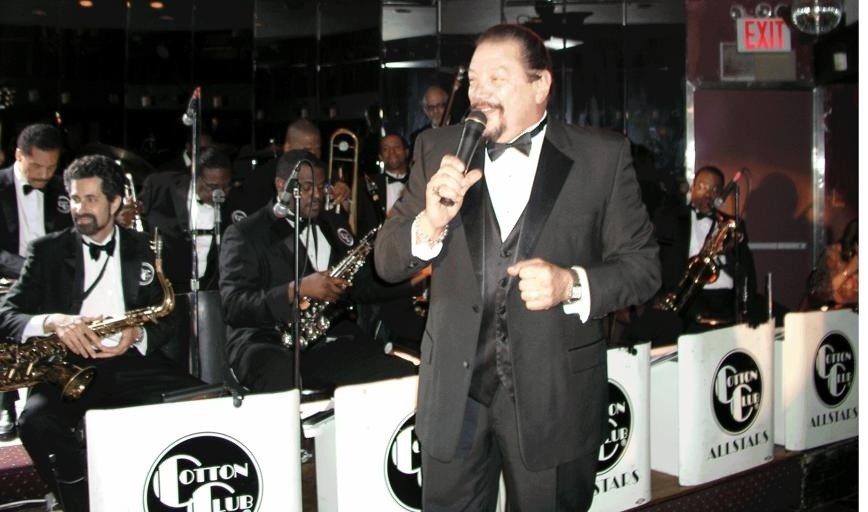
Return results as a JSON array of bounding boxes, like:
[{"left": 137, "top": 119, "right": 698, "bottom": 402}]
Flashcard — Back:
[{"left": 565, "top": 267, "right": 584, "bottom": 305}]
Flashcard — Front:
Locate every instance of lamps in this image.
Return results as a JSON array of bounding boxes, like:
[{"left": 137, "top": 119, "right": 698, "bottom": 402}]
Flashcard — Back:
[
  {"left": 790, "top": 1, "right": 845, "bottom": 36},
  {"left": 513, "top": 0, "right": 597, "bottom": 56},
  {"left": 726, "top": 1, "right": 790, "bottom": 22}
]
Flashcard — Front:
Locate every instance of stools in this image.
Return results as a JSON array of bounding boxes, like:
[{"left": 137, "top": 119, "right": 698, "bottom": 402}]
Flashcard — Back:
[{"left": 1, "top": 433, "right": 62, "bottom": 511}]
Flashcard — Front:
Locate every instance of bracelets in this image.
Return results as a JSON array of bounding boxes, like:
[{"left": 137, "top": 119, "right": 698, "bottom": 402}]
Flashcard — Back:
[
  {"left": 839, "top": 269, "right": 849, "bottom": 279},
  {"left": 413, "top": 208, "right": 452, "bottom": 249}
]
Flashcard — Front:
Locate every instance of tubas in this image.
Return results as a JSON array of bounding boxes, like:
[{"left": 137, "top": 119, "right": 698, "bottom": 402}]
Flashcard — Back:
[{"left": 320, "top": 128, "right": 360, "bottom": 238}]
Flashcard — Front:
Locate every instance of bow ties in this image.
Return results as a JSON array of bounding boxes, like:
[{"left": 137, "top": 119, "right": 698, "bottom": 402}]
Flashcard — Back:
[
  {"left": 297, "top": 219, "right": 320, "bottom": 234},
  {"left": 387, "top": 175, "right": 409, "bottom": 185},
  {"left": 695, "top": 212, "right": 714, "bottom": 222},
  {"left": 22, "top": 184, "right": 46, "bottom": 195},
  {"left": 89, "top": 238, "right": 117, "bottom": 261},
  {"left": 484, "top": 131, "right": 533, "bottom": 162}
]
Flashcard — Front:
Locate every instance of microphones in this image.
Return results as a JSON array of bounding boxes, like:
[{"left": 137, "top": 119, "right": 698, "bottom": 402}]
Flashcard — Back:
[
  {"left": 182, "top": 91, "right": 200, "bottom": 125},
  {"left": 212, "top": 189, "right": 223, "bottom": 244},
  {"left": 436, "top": 110, "right": 487, "bottom": 205},
  {"left": 273, "top": 163, "right": 303, "bottom": 218},
  {"left": 713, "top": 171, "right": 743, "bottom": 206}
]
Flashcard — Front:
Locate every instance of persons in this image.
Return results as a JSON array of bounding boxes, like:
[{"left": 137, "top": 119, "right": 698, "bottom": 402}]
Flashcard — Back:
[
  {"left": 214, "top": 148, "right": 418, "bottom": 392},
  {"left": 821, "top": 249, "right": 861, "bottom": 308},
  {"left": 1, "top": 155, "right": 176, "bottom": 511},
  {"left": 370, "top": 22, "right": 665, "bottom": 512}
]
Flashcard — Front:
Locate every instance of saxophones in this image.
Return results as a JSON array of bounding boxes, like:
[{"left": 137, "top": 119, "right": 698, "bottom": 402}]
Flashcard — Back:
[
  {"left": 653, "top": 202, "right": 737, "bottom": 318},
  {"left": 1, "top": 225, "right": 177, "bottom": 405},
  {"left": 273, "top": 172, "right": 389, "bottom": 353}
]
